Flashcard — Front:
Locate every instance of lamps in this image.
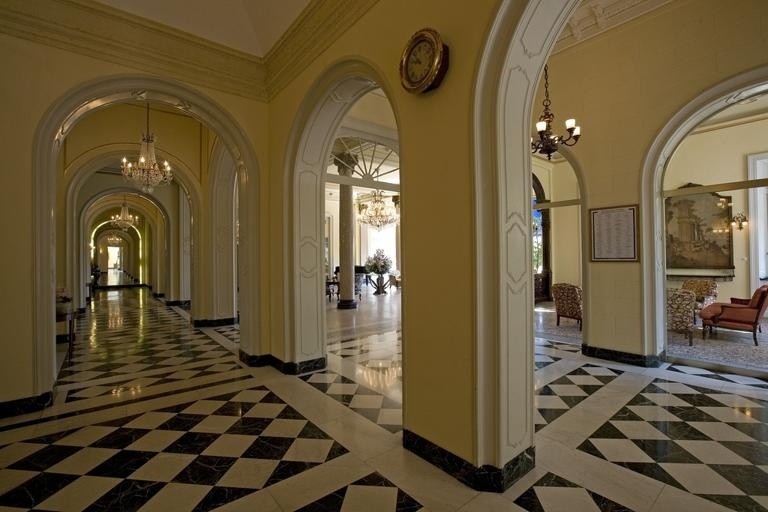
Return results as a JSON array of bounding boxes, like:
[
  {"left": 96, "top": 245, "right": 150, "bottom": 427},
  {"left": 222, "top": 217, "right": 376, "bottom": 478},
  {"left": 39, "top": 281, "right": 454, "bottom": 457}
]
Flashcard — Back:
[
  {"left": 359, "top": 168, "right": 400, "bottom": 230},
  {"left": 531, "top": 63, "right": 582, "bottom": 162},
  {"left": 113, "top": 193, "right": 139, "bottom": 232},
  {"left": 120, "top": 102, "right": 173, "bottom": 196}
]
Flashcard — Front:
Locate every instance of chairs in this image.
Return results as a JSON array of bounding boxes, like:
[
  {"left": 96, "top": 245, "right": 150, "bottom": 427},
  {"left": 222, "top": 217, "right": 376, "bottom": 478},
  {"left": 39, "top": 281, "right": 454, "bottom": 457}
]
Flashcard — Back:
[
  {"left": 552, "top": 283, "right": 583, "bottom": 332},
  {"left": 390, "top": 273, "right": 402, "bottom": 290},
  {"left": 667, "top": 279, "right": 767, "bottom": 347}
]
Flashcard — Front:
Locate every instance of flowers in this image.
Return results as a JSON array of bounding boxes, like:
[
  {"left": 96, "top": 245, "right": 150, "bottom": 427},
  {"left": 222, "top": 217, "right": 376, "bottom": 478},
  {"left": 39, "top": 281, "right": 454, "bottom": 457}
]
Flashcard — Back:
[{"left": 367, "top": 248, "right": 393, "bottom": 274}]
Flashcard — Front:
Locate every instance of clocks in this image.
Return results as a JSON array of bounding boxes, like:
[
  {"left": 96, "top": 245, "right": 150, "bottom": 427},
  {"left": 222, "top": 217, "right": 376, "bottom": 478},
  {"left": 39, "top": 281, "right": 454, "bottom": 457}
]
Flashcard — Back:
[{"left": 399, "top": 27, "right": 449, "bottom": 97}]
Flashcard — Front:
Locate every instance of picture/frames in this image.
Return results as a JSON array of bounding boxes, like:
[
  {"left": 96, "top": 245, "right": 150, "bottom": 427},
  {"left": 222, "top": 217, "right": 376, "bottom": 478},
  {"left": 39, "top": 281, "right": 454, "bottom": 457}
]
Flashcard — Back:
[
  {"left": 588, "top": 204, "right": 640, "bottom": 264},
  {"left": 664, "top": 183, "right": 736, "bottom": 269}
]
